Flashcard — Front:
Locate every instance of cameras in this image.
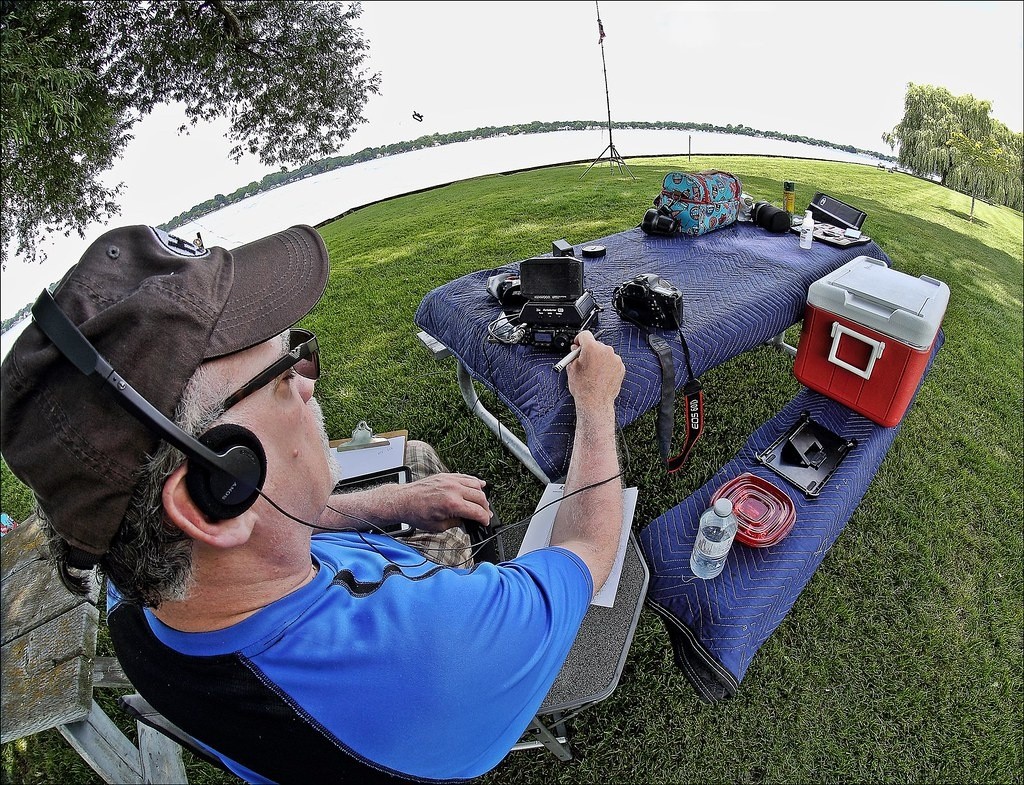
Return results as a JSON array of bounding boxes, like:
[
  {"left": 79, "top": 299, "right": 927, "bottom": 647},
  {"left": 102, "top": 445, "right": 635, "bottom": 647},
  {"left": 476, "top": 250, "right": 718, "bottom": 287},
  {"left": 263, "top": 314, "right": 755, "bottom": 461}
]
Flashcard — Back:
[
  {"left": 616, "top": 273, "right": 684, "bottom": 330},
  {"left": 30, "top": 288, "right": 267, "bottom": 520}
]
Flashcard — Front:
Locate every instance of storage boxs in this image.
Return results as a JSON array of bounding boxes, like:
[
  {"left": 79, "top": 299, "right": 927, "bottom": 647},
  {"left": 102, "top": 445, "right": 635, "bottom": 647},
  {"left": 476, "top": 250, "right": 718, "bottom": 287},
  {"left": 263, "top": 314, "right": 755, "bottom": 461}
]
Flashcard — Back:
[
  {"left": 552, "top": 239, "right": 573, "bottom": 258},
  {"left": 794, "top": 253, "right": 949, "bottom": 427},
  {"left": 808, "top": 191, "right": 867, "bottom": 230}
]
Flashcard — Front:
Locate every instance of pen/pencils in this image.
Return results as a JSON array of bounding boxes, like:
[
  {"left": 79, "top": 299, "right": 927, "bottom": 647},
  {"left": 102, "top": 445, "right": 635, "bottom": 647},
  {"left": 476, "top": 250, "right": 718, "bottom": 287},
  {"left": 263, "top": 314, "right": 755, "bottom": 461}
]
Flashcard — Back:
[{"left": 552, "top": 327, "right": 607, "bottom": 373}]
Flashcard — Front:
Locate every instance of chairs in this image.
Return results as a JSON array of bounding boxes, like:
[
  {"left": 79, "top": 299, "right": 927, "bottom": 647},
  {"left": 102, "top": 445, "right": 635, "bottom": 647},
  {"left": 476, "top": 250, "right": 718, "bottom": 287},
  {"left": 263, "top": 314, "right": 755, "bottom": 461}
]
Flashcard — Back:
[{"left": 121, "top": 514, "right": 572, "bottom": 779}]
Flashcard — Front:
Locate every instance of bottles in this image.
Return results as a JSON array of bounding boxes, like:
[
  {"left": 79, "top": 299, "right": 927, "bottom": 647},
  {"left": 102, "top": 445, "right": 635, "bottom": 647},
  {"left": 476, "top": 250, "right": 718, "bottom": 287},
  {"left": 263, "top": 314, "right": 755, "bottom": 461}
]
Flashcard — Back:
[
  {"left": 783, "top": 181, "right": 795, "bottom": 214},
  {"left": 800, "top": 211, "right": 814, "bottom": 249},
  {"left": 690, "top": 498, "right": 738, "bottom": 578}
]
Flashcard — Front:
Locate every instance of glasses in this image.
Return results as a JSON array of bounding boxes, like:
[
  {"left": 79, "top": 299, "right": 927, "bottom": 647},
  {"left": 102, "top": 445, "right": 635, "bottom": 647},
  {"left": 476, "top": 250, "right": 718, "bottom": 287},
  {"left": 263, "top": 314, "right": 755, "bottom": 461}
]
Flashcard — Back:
[{"left": 221, "top": 327, "right": 321, "bottom": 414}]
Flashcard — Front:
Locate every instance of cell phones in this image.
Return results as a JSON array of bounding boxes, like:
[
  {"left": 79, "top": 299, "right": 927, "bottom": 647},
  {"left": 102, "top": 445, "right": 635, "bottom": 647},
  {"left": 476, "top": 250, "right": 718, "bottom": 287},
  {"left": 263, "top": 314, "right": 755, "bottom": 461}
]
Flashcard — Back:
[{"left": 844, "top": 227, "right": 862, "bottom": 239}]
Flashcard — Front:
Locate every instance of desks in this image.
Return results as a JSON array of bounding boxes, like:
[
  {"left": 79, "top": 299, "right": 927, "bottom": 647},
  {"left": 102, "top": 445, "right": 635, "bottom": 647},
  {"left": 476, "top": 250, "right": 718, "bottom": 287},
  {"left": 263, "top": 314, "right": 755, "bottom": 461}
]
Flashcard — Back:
[
  {"left": 646, "top": 327, "right": 946, "bottom": 707},
  {"left": 415, "top": 223, "right": 886, "bottom": 479},
  {"left": 529, "top": 527, "right": 650, "bottom": 715},
  {"left": 0, "top": 510, "right": 188, "bottom": 785}
]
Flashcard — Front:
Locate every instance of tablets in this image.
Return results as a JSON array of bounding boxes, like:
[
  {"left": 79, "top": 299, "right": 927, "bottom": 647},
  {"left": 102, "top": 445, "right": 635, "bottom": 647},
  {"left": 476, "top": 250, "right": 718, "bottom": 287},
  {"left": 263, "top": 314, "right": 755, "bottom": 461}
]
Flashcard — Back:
[{"left": 330, "top": 465, "right": 415, "bottom": 538}]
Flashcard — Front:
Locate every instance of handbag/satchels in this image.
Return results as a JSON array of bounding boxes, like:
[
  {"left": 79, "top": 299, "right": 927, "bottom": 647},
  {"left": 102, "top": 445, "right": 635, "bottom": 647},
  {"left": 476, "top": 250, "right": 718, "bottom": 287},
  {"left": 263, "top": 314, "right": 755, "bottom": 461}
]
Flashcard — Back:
[{"left": 654, "top": 168, "right": 742, "bottom": 238}]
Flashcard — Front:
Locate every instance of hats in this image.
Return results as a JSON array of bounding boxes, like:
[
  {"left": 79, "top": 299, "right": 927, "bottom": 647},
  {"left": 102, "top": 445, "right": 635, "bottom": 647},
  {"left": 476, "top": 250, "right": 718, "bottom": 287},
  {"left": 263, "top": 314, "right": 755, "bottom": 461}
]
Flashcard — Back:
[{"left": 1, "top": 224, "right": 330, "bottom": 570}]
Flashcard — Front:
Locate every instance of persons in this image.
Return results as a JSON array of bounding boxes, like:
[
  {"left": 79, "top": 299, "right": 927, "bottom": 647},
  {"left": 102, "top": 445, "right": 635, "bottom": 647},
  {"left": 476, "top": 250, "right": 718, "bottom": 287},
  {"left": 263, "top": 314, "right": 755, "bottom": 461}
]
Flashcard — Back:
[{"left": 0, "top": 222, "right": 626, "bottom": 785}]
